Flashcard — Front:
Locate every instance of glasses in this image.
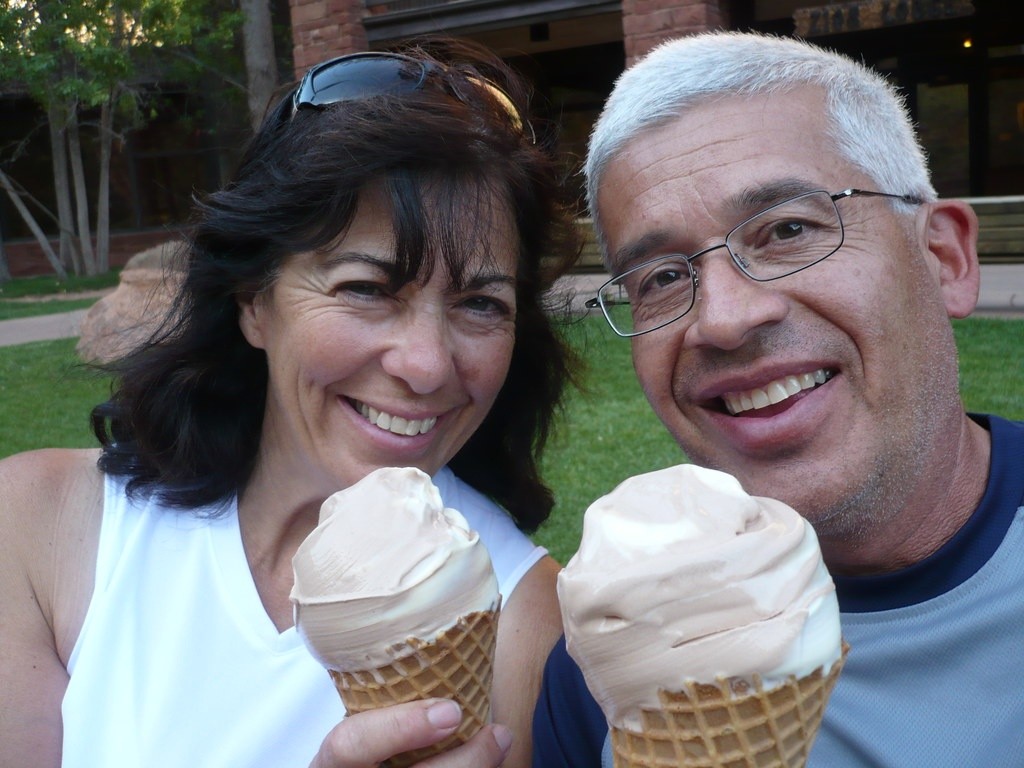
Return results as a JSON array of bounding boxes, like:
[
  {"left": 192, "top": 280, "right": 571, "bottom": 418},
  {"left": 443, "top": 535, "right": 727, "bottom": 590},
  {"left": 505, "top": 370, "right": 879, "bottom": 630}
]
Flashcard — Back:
[
  {"left": 284, "top": 52, "right": 537, "bottom": 146},
  {"left": 585, "top": 187, "right": 927, "bottom": 338}
]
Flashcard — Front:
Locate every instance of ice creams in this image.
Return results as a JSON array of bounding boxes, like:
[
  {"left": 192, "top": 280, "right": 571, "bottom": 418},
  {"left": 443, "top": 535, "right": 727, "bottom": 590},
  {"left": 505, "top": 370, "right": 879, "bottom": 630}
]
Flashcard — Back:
[
  {"left": 288, "top": 465, "right": 504, "bottom": 768},
  {"left": 555, "top": 463, "right": 849, "bottom": 768}
]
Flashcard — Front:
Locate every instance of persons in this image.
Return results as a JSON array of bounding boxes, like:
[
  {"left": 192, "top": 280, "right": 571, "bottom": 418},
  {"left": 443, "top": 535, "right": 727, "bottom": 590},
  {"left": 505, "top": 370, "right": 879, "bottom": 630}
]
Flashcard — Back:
[
  {"left": 527, "top": 30, "right": 1022, "bottom": 767},
  {"left": 0, "top": 37, "right": 584, "bottom": 768}
]
